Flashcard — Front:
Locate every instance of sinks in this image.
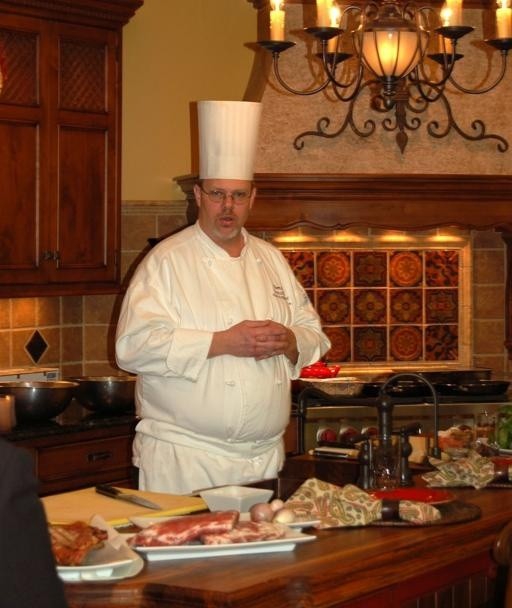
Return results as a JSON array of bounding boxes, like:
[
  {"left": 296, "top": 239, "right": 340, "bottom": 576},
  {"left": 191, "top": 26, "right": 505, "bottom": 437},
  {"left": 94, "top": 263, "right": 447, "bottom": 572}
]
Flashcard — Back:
[{"left": 363, "top": 485, "right": 458, "bottom": 504}]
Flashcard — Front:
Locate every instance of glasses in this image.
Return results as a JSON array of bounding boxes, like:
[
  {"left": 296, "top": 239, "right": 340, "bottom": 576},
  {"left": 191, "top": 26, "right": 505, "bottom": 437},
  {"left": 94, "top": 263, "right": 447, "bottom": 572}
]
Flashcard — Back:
[{"left": 200, "top": 187, "right": 250, "bottom": 202}]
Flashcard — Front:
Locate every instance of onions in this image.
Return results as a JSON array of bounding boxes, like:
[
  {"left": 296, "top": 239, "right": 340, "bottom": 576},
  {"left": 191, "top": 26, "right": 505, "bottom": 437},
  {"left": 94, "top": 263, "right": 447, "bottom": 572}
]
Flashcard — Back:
[{"left": 250, "top": 498, "right": 294, "bottom": 525}]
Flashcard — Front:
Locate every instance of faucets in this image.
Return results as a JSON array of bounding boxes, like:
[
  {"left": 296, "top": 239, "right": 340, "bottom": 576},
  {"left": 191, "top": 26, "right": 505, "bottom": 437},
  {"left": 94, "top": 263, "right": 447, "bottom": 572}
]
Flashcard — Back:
[{"left": 377, "top": 373, "right": 439, "bottom": 459}]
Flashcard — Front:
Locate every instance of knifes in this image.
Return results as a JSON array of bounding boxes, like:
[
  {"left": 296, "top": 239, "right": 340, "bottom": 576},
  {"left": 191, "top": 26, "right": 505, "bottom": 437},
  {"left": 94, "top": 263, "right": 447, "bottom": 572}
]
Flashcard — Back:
[{"left": 96, "top": 480, "right": 166, "bottom": 512}]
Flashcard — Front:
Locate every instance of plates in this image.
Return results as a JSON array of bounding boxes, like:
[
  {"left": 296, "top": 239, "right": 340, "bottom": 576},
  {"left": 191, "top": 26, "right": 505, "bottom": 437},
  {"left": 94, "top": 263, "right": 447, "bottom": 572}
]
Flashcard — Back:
[
  {"left": 371, "top": 488, "right": 457, "bottom": 507},
  {"left": 52, "top": 523, "right": 138, "bottom": 572},
  {"left": 362, "top": 378, "right": 511, "bottom": 401},
  {"left": 127, "top": 513, "right": 320, "bottom": 531},
  {"left": 117, "top": 532, "right": 318, "bottom": 559},
  {"left": 479, "top": 437, "right": 511, "bottom": 454}
]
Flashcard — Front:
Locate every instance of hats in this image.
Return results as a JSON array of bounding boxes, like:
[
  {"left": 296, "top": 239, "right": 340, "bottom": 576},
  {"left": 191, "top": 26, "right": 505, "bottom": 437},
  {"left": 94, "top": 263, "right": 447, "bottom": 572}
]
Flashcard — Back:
[{"left": 197, "top": 100, "right": 262, "bottom": 181}]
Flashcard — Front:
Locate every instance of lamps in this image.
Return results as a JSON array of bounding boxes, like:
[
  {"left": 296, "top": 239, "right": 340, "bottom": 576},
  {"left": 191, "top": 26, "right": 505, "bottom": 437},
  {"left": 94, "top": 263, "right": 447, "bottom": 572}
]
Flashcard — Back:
[{"left": 246, "top": 0, "right": 512, "bottom": 154}]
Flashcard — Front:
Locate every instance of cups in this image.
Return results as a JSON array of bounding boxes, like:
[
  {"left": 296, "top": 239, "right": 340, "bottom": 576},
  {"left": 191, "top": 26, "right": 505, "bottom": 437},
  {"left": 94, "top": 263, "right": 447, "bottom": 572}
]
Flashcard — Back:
[{"left": 367, "top": 435, "right": 401, "bottom": 490}]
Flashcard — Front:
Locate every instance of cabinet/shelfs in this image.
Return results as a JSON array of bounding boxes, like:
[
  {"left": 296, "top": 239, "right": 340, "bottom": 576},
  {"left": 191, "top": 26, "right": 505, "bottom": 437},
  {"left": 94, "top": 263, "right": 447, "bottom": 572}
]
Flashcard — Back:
[
  {"left": 0, "top": 0, "right": 141, "bottom": 299},
  {"left": 34, "top": 424, "right": 138, "bottom": 498}
]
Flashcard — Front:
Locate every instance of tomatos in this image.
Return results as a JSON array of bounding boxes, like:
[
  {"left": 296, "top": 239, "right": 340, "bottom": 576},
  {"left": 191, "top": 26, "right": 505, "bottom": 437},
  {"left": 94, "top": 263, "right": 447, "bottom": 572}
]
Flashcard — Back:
[{"left": 300, "top": 361, "right": 340, "bottom": 379}]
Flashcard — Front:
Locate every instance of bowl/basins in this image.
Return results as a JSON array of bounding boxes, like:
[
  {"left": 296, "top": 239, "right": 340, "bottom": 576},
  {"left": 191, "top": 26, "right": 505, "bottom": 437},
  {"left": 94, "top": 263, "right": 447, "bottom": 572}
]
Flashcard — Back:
[
  {"left": 73, "top": 375, "right": 138, "bottom": 414},
  {"left": 0, "top": 379, "right": 78, "bottom": 418},
  {"left": 198, "top": 484, "right": 274, "bottom": 511}
]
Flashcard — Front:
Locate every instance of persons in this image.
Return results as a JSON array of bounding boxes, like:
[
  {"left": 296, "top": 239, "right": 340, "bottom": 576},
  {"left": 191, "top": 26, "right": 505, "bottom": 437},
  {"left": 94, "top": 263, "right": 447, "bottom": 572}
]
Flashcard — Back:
[
  {"left": 113, "top": 180, "right": 333, "bottom": 495},
  {"left": 0, "top": 440, "right": 68, "bottom": 608}
]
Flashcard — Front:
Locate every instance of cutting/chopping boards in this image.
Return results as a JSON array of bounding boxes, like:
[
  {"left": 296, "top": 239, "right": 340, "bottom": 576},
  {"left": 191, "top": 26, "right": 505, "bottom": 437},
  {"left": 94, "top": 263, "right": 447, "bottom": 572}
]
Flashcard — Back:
[{"left": 40, "top": 485, "right": 209, "bottom": 527}]
